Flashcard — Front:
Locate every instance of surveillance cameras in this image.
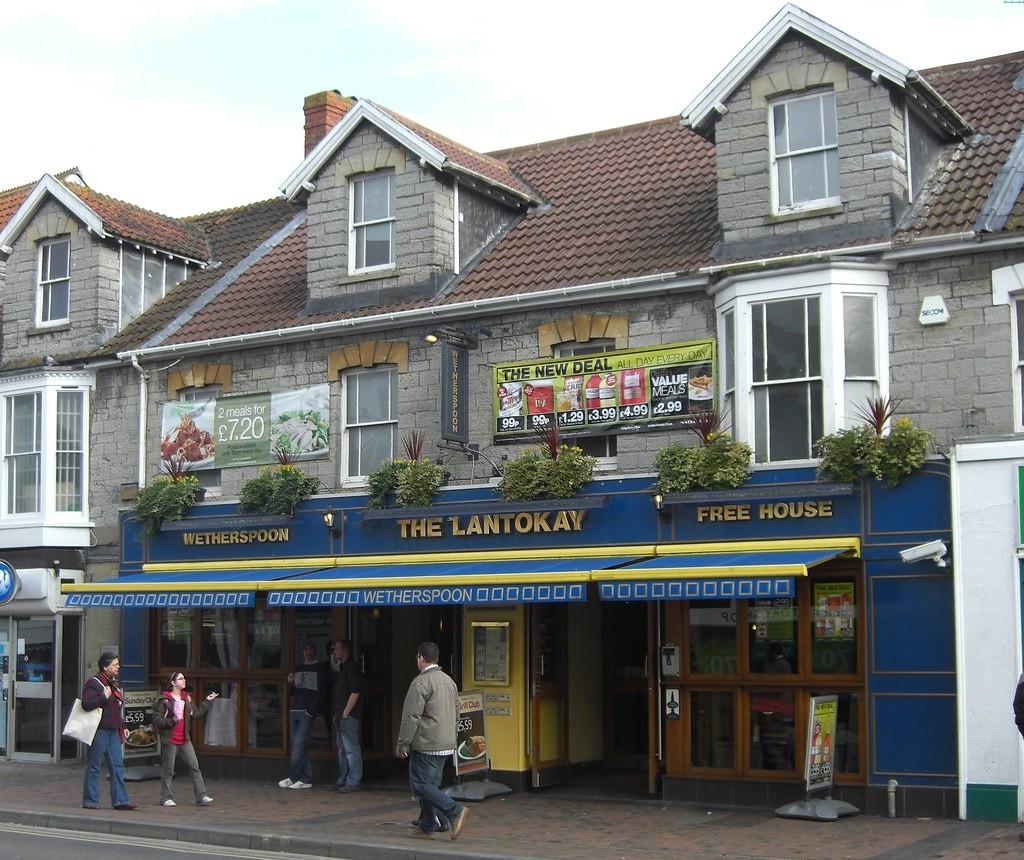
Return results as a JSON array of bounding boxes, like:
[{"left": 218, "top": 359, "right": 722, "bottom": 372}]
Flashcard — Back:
[{"left": 899, "top": 540, "right": 947, "bottom": 564}]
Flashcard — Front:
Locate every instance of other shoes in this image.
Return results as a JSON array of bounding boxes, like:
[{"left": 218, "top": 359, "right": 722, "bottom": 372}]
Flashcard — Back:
[
  {"left": 83, "top": 804, "right": 101, "bottom": 809},
  {"left": 1019, "top": 832, "right": 1024, "bottom": 842},
  {"left": 163, "top": 798, "right": 177, "bottom": 807},
  {"left": 196, "top": 796, "right": 214, "bottom": 804},
  {"left": 412, "top": 819, "right": 449, "bottom": 832},
  {"left": 114, "top": 802, "right": 139, "bottom": 810},
  {"left": 327, "top": 782, "right": 344, "bottom": 791},
  {"left": 338, "top": 784, "right": 362, "bottom": 793}
]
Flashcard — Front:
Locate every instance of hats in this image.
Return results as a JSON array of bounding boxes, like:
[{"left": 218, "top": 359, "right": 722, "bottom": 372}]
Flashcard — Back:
[{"left": 302, "top": 640, "right": 317, "bottom": 657}]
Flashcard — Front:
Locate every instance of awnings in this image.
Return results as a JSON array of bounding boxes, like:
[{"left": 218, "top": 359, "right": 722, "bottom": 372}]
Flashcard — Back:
[
  {"left": 259, "top": 545, "right": 655, "bottom": 606},
  {"left": 591, "top": 537, "right": 860, "bottom": 599},
  {"left": 61, "top": 558, "right": 336, "bottom": 608}
]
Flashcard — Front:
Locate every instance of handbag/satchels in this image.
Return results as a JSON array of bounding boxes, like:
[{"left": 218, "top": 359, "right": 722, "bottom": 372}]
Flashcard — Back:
[{"left": 62, "top": 676, "right": 107, "bottom": 747}]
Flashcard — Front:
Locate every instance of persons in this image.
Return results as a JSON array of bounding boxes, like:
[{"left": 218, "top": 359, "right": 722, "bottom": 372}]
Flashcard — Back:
[
  {"left": 330, "top": 639, "right": 363, "bottom": 793},
  {"left": 82, "top": 652, "right": 140, "bottom": 810},
  {"left": 279, "top": 644, "right": 328, "bottom": 789},
  {"left": 395, "top": 643, "right": 468, "bottom": 840},
  {"left": 1013, "top": 672, "right": 1024, "bottom": 841},
  {"left": 152, "top": 672, "right": 219, "bottom": 807}
]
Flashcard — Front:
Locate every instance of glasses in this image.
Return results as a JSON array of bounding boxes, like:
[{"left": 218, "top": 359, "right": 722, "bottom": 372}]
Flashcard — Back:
[
  {"left": 110, "top": 662, "right": 120, "bottom": 666},
  {"left": 177, "top": 677, "right": 185, "bottom": 681},
  {"left": 416, "top": 656, "right": 421, "bottom": 659}
]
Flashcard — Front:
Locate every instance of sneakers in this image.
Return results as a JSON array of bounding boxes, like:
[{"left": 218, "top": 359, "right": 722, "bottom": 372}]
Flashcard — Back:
[
  {"left": 407, "top": 826, "right": 435, "bottom": 840},
  {"left": 288, "top": 780, "right": 312, "bottom": 788},
  {"left": 450, "top": 806, "right": 470, "bottom": 840},
  {"left": 278, "top": 777, "right": 294, "bottom": 788}
]
